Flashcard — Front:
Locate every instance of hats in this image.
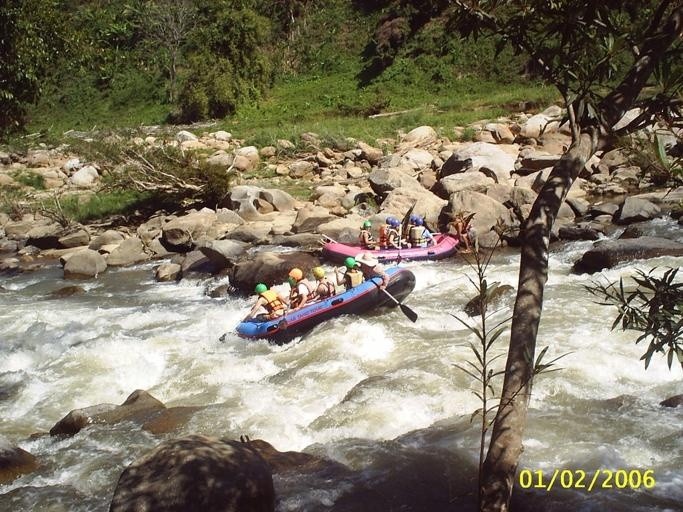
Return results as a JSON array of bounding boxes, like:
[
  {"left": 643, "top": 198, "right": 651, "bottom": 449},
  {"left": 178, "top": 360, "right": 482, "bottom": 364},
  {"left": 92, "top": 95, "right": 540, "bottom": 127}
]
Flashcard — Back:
[
  {"left": 355, "top": 253, "right": 378, "bottom": 267},
  {"left": 312, "top": 267, "right": 325, "bottom": 280},
  {"left": 344, "top": 257, "right": 355, "bottom": 268}
]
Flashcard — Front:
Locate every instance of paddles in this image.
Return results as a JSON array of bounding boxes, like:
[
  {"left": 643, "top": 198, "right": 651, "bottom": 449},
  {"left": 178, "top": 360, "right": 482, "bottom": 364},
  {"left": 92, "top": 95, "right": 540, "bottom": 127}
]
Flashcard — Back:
[
  {"left": 355, "top": 264, "right": 418, "bottom": 323},
  {"left": 397, "top": 235, "right": 401, "bottom": 265}
]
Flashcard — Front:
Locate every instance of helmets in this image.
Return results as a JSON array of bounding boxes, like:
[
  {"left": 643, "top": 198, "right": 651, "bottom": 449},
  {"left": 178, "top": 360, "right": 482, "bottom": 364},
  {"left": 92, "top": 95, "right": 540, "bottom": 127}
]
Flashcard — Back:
[
  {"left": 411, "top": 217, "right": 423, "bottom": 225},
  {"left": 386, "top": 217, "right": 400, "bottom": 227},
  {"left": 254, "top": 284, "right": 267, "bottom": 293},
  {"left": 288, "top": 268, "right": 302, "bottom": 287},
  {"left": 363, "top": 221, "right": 372, "bottom": 227}
]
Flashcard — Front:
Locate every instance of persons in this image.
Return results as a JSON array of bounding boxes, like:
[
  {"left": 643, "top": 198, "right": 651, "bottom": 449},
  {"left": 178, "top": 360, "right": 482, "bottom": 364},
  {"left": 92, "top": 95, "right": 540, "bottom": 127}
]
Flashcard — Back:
[
  {"left": 378, "top": 217, "right": 394, "bottom": 250},
  {"left": 387, "top": 219, "right": 412, "bottom": 249},
  {"left": 447, "top": 214, "right": 479, "bottom": 254},
  {"left": 288, "top": 267, "right": 318, "bottom": 310},
  {"left": 411, "top": 217, "right": 437, "bottom": 248},
  {"left": 333, "top": 257, "right": 363, "bottom": 290},
  {"left": 308, "top": 266, "right": 336, "bottom": 298},
  {"left": 240, "top": 282, "right": 289, "bottom": 324},
  {"left": 358, "top": 221, "right": 379, "bottom": 249},
  {"left": 355, "top": 251, "right": 391, "bottom": 291},
  {"left": 405, "top": 216, "right": 419, "bottom": 245}
]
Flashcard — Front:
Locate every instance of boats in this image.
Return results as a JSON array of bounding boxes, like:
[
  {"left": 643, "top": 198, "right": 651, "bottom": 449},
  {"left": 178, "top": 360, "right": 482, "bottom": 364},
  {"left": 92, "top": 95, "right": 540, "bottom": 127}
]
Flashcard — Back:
[
  {"left": 235, "top": 266, "right": 416, "bottom": 345},
  {"left": 323, "top": 233, "right": 460, "bottom": 265}
]
